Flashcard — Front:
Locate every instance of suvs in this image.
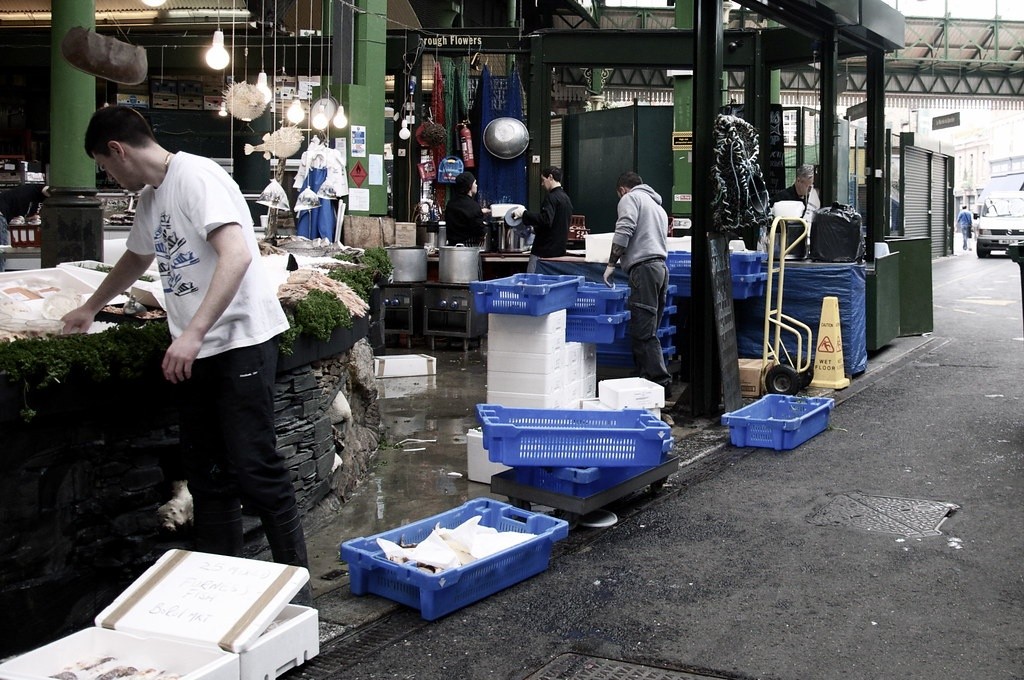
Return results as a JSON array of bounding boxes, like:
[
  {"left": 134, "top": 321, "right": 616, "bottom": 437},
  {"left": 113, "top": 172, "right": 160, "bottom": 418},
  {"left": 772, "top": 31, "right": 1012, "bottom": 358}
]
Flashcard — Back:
[{"left": 973, "top": 190, "right": 1024, "bottom": 258}]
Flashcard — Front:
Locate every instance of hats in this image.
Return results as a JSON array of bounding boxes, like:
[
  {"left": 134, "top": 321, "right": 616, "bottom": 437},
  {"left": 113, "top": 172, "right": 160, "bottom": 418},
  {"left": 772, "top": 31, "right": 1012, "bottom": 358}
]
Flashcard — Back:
[{"left": 454, "top": 172, "right": 475, "bottom": 194}]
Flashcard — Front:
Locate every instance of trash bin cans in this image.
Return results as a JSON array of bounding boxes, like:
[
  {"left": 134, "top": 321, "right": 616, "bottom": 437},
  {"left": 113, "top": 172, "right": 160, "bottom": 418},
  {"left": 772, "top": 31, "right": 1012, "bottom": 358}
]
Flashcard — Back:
[{"left": 865, "top": 235, "right": 933, "bottom": 349}]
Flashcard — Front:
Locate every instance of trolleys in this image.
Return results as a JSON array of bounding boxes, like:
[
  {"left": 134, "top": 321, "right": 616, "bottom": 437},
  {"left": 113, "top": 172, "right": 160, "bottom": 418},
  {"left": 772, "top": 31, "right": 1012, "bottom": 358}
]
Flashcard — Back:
[{"left": 737, "top": 216, "right": 814, "bottom": 400}]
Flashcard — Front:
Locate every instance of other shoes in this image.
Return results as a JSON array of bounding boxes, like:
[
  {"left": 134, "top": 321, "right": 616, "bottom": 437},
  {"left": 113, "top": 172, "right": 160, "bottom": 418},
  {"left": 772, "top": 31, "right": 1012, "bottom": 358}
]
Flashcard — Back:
[{"left": 963, "top": 246, "right": 967, "bottom": 250}]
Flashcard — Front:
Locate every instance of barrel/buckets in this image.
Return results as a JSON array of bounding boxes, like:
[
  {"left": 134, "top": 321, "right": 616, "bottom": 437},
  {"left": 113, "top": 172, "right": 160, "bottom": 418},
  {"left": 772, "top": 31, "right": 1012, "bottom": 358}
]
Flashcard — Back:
[
  {"left": 439, "top": 243, "right": 485, "bottom": 283},
  {"left": 385, "top": 247, "right": 428, "bottom": 282}
]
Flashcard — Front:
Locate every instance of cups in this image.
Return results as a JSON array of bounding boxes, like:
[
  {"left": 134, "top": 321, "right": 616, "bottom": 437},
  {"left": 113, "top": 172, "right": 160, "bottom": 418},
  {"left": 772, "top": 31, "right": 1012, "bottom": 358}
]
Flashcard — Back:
[{"left": 260, "top": 215, "right": 268, "bottom": 227}]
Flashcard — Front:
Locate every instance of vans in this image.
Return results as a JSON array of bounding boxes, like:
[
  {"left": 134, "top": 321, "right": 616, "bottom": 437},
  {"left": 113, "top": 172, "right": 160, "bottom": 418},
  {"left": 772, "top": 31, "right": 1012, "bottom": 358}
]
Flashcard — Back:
[{"left": 0, "top": 154, "right": 27, "bottom": 193}]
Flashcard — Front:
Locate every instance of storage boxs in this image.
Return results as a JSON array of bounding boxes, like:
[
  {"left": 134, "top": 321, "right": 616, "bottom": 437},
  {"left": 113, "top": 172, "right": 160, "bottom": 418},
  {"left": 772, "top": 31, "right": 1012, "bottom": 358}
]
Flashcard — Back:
[
  {"left": 338, "top": 232, "right": 835, "bottom": 620},
  {"left": 0, "top": 259, "right": 165, "bottom": 305},
  {"left": 0, "top": 548, "right": 319, "bottom": 680}
]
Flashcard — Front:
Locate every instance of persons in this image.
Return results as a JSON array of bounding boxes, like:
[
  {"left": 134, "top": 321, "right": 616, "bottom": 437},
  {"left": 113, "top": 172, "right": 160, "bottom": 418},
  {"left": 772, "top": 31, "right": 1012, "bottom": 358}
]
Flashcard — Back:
[
  {"left": 958, "top": 205, "right": 971, "bottom": 250},
  {"left": 604, "top": 172, "right": 671, "bottom": 401},
  {"left": 446, "top": 172, "right": 486, "bottom": 282},
  {"left": 58, "top": 104, "right": 312, "bottom": 605},
  {"left": 515, "top": 166, "right": 574, "bottom": 273},
  {"left": 769, "top": 164, "right": 815, "bottom": 207}
]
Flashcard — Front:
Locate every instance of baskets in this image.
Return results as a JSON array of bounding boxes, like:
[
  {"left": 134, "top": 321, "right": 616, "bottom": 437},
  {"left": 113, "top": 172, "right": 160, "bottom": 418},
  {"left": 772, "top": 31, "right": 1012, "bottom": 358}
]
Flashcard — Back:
[
  {"left": 666, "top": 251, "right": 767, "bottom": 275},
  {"left": 339, "top": 497, "right": 570, "bottom": 622},
  {"left": 566, "top": 281, "right": 678, "bottom": 370},
  {"left": 721, "top": 393, "right": 834, "bottom": 451},
  {"left": 513, "top": 433, "right": 675, "bottom": 499},
  {"left": 668, "top": 274, "right": 767, "bottom": 300},
  {"left": 475, "top": 403, "right": 672, "bottom": 467},
  {"left": 469, "top": 273, "right": 586, "bottom": 317}
]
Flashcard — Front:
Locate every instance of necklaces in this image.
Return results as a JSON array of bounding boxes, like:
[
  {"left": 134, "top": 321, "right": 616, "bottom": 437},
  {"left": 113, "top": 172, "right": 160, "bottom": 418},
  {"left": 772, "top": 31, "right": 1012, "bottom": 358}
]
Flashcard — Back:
[{"left": 164, "top": 152, "right": 173, "bottom": 173}]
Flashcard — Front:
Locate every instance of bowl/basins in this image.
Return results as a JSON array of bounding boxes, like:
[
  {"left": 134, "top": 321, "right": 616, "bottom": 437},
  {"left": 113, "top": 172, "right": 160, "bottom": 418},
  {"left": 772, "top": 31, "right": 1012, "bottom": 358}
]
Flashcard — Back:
[{"left": 483, "top": 118, "right": 529, "bottom": 159}]
